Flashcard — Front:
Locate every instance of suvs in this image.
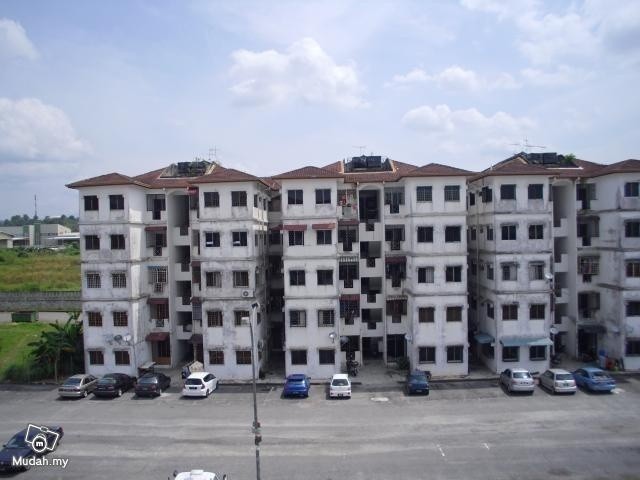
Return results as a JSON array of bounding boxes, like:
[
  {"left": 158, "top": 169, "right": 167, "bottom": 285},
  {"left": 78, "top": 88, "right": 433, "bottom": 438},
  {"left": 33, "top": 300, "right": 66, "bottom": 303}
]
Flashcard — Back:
[
  {"left": 404, "top": 370, "right": 430, "bottom": 395},
  {"left": 179, "top": 371, "right": 220, "bottom": 398}
]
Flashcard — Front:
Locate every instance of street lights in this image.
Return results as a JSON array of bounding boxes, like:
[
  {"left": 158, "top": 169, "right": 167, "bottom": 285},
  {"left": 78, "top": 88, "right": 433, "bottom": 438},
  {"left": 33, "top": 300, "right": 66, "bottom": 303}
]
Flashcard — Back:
[{"left": 238, "top": 299, "right": 263, "bottom": 480}]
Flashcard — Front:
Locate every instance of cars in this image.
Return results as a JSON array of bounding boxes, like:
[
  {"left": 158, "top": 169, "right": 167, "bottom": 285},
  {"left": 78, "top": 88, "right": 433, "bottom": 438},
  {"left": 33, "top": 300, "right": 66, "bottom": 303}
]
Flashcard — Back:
[
  {"left": 58, "top": 373, "right": 98, "bottom": 399},
  {"left": 92, "top": 372, "right": 138, "bottom": 400},
  {"left": 282, "top": 373, "right": 313, "bottom": 398},
  {"left": 498, "top": 366, "right": 617, "bottom": 395},
  {"left": 0, "top": 423, "right": 66, "bottom": 475},
  {"left": 135, "top": 372, "right": 171, "bottom": 397},
  {"left": 166, "top": 469, "right": 227, "bottom": 480}
]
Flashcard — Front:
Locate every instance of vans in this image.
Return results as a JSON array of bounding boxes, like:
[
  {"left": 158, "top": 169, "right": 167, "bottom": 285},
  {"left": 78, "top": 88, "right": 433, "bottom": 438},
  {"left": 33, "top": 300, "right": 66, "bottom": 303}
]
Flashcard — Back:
[{"left": 328, "top": 373, "right": 352, "bottom": 398}]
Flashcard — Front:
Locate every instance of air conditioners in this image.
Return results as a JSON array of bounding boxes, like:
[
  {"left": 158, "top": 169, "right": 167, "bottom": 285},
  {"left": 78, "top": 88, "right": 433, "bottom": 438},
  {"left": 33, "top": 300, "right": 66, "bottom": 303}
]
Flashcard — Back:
[
  {"left": 259, "top": 339, "right": 270, "bottom": 350},
  {"left": 242, "top": 290, "right": 256, "bottom": 298}
]
